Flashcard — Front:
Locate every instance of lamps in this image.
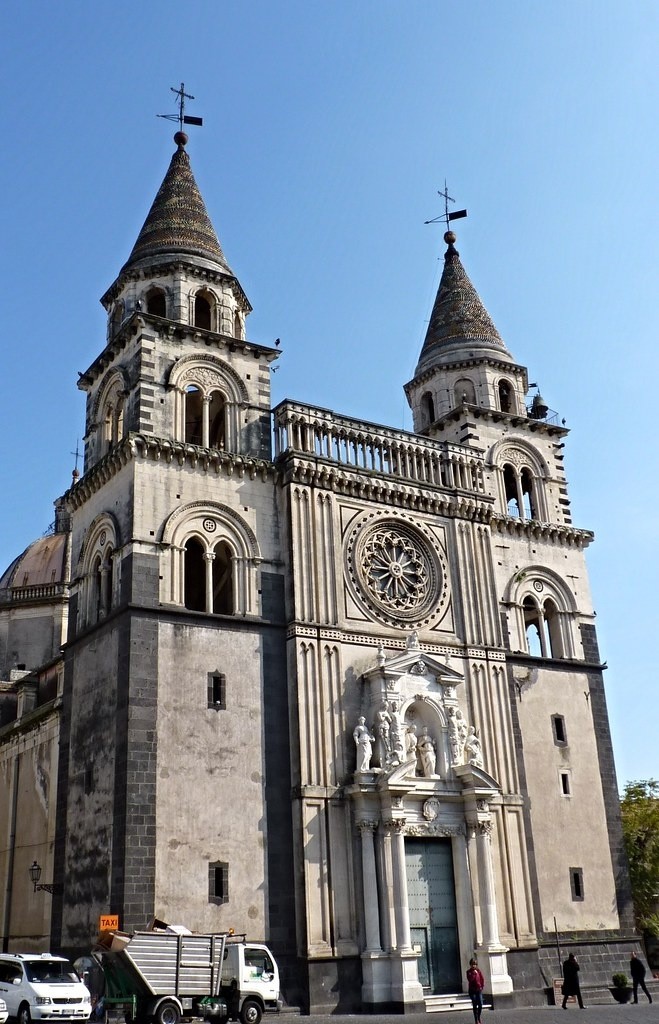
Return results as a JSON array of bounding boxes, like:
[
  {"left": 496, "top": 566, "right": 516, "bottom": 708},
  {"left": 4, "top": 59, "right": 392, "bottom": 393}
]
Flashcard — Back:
[{"left": 28, "top": 861, "right": 53, "bottom": 894}]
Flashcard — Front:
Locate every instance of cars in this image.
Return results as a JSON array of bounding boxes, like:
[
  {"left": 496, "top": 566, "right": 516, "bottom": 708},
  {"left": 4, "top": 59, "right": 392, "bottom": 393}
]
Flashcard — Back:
[{"left": 0, "top": 953, "right": 92, "bottom": 1024}]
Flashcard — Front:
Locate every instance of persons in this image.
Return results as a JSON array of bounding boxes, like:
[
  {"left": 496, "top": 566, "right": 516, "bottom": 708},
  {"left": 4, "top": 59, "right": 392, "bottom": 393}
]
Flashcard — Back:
[
  {"left": 377, "top": 701, "right": 405, "bottom": 766},
  {"left": 418, "top": 726, "right": 437, "bottom": 775},
  {"left": 449, "top": 706, "right": 466, "bottom": 757},
  {"left": 353, "top": 717, "right": 375, "bottom": 770},
  {"left": 561, "top": 954, "right": 589, "bottom": 1009},
  {"left": 629, "top": 952, "right": 652, "bottom": 1003},
  {"left": 466, "top": 958, "right": 485, "bottom": 1023},
  {"left": 465, "top": 726, "right": 481, "bottom": 760},
  {"left": 406, "top": 725, "right": 417, "bottom": 775}
]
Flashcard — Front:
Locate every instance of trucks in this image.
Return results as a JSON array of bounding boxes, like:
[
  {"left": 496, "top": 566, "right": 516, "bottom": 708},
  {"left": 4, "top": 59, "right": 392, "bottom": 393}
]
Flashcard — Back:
[{"left": 104, "top": 928, "right": 283, "bottom": 1024}]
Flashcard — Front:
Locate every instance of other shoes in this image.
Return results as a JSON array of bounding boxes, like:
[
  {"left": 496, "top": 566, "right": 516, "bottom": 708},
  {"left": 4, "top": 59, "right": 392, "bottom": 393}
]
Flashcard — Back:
[
  {"left": 562, "top": 1006, "right": 568, "bottom": 1009},
  {"left": 631, "top": 1001, "right": 638, "bottom": 1004},
  {"left": 474, "top": 1019, "right": 482, "bottom": 1024},
  {"left": 649, "top": 998, "right": 652, "bottom": 1004},
  {"left": 581, "top": 1006, "right": 588, "bottom": 1009}
]
